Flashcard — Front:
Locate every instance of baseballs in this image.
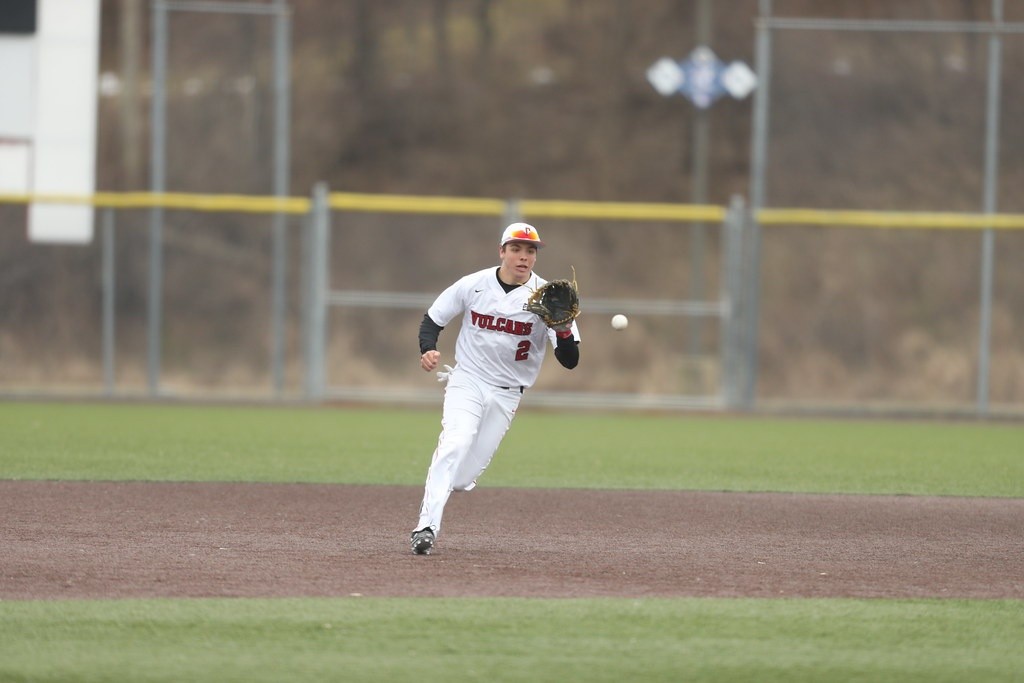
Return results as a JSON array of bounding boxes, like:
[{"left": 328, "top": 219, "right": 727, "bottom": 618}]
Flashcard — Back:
[{"left": 610, "top": 314, "right": 629, "bottom": 331}]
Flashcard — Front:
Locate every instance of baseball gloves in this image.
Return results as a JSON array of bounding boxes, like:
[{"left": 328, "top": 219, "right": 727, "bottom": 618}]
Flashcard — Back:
[{"left": 523, "top": 278, "right": 581, "bottom": 333}]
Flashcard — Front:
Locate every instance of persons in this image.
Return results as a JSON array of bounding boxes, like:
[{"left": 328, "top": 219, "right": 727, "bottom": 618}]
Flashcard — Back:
[{"left": 409, "top": 223, "right": 581, "bottom": 554}]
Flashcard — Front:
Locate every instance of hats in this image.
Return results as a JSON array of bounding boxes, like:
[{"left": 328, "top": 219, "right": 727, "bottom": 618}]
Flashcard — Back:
[{"left": 501, "top": 220, "right": 546, "bottom": 253}]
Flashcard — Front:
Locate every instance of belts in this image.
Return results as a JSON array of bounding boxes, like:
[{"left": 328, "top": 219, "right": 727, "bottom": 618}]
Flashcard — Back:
[{"left": 501, "top": 386, "right": 524, "bottom": 394}]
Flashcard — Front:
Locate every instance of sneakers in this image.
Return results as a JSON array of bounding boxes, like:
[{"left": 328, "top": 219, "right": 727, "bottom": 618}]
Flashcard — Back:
[{"left": 413, "top": 529, "right": 435, "bottom": 554}]
